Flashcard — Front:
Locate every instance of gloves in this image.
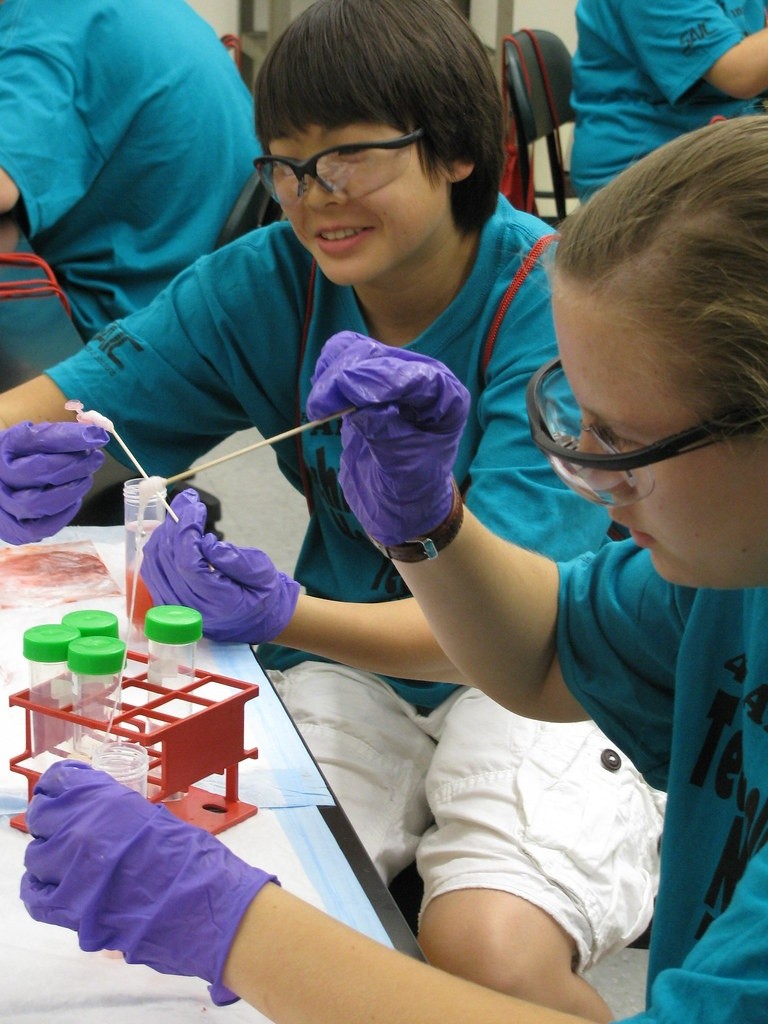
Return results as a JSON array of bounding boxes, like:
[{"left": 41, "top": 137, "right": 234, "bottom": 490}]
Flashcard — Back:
[
  {"left": 20, "top": 758, "right": 285, "bottom": 1008},
  {"left": 305, "top": 331, "right": 470, "bottom": 550},
  {"left": 140, "top": 488, "right": 299, "bottom": 646},
  {"left": 0, "top": 421, "right": 110, "bottom": 545}
]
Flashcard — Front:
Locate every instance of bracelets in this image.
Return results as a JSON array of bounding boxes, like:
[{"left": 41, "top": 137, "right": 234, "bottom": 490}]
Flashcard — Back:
[{"left": 366, "top": 473, "right": 464, "bottom": 565}]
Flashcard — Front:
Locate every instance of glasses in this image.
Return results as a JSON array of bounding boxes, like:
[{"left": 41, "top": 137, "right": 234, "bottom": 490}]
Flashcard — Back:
[
  {"left": 526, "top": 356, "right": 768, "bottom": 509},
  {"left": 252, "top": 127, "right": 425, "bottom": 205}
]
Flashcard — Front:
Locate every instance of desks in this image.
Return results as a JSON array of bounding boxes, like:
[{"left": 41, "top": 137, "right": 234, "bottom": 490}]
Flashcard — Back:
[{"left": 0, "top": 207, "right": 427, "bottom": 1024}]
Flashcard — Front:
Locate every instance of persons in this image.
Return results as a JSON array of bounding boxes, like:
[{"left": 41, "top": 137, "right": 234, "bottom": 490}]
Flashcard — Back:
[
  {"left": 0, "top": 0, "right": 262, "bottom": 343},
  {"left": 19, "top": 114, "right": 768, "bottom": 1024},
  {"left": 568, "top": 0, "right": 768, "bottom": 205},
  {"left": 0, "top": 0, "right": 668, "bottom": 1024}
]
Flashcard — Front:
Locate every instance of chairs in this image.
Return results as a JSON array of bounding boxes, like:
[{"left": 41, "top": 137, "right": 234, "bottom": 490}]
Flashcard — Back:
[{"left": 505, "top": 27, "right": 573, "bottom": 230}]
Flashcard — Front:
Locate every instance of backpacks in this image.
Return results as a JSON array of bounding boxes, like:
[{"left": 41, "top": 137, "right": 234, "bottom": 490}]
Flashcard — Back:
[{"left": 497, "top": 28, "right": 566, "bottom": 215}]
